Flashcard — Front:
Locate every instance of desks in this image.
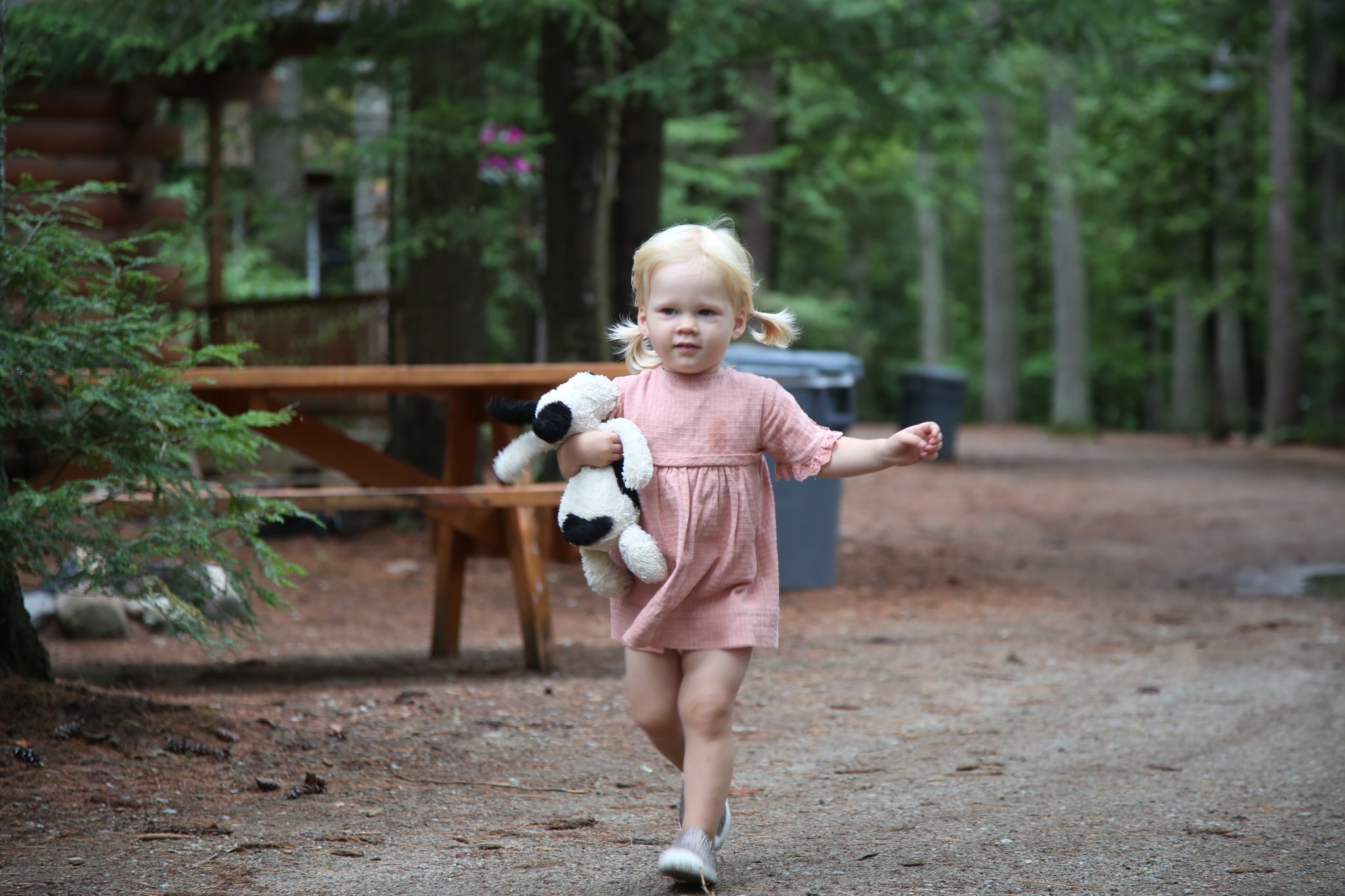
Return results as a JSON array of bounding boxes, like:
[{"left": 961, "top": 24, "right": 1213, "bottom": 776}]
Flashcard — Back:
[{"left": 1, "top": 360, "right": 640, "bottom": 668}]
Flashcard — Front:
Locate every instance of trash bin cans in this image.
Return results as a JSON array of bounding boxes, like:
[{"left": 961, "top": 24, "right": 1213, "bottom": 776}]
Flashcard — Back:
[
  {"left": 899, "top": 363, "right": 968, "bottom": 464},
  {"left": 722, "top": 345, "right": 862, "bottom": 593}
]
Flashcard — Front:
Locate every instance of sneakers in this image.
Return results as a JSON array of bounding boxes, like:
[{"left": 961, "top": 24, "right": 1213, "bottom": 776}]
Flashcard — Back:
[
  {"left": 679, "top": 772, "right": 731, "bottom": 850},
  {"left": 656, "top": 827, "right": 717, "bottom": 885}
]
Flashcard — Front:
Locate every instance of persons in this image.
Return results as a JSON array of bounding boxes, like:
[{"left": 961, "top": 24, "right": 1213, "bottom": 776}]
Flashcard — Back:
[{"left": 557, "top": 225, "right": 942, "bottom": 884}]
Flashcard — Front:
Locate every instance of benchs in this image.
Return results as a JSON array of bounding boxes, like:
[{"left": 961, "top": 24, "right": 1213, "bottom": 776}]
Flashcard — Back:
[{"left": 1, "top": 476, "right": 588, "bottom": 516}]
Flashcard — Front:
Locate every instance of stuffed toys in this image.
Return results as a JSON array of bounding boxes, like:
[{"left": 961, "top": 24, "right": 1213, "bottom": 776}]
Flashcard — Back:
[{"left": 482, "top": 369, "right": 667, "bottom": 600}]
